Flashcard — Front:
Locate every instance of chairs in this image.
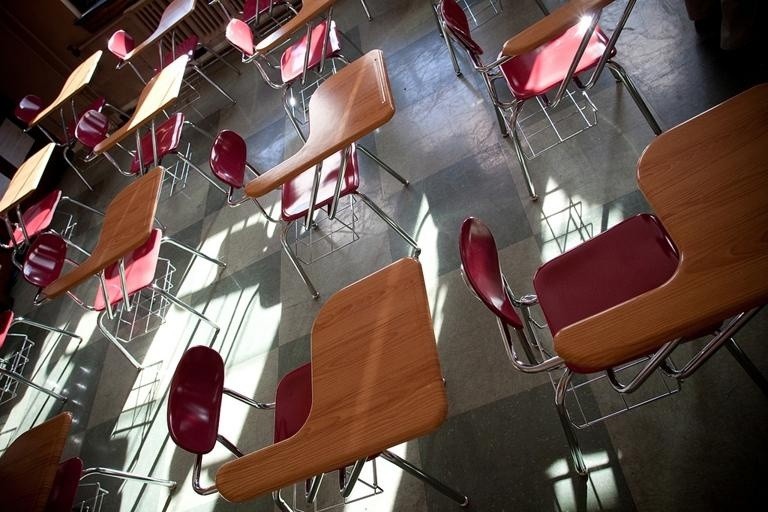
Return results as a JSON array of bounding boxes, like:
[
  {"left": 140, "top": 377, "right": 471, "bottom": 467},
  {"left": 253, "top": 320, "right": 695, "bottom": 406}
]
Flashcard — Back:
[{"left": 0, "top": 0, "right": 768, "bottom": 511}]
